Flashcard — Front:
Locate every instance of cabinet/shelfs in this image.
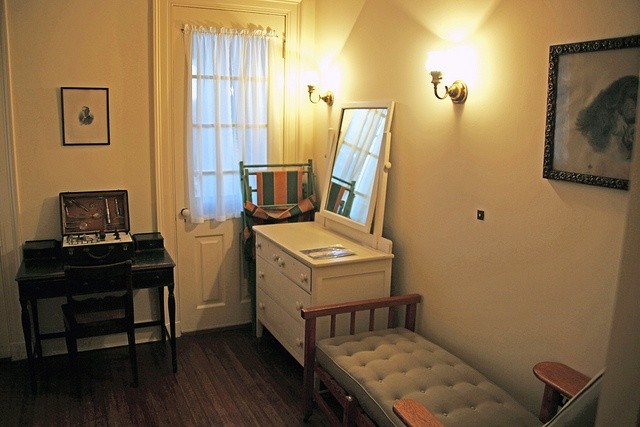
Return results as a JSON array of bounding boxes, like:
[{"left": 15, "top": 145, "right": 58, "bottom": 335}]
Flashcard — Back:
[{"left": 251, "top": 223, "right": 394, "bottom": 401}]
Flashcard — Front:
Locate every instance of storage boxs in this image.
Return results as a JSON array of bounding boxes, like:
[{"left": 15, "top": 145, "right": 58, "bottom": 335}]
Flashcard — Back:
[{"left": 60, "top": 188, "right": 136, "bottom": 259}]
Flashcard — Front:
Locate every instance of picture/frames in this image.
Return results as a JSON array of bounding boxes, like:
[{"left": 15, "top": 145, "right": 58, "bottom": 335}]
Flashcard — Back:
[
  {"left": 541, "top": 34, "right": 640, "bottom": 193},
  {"left": 60, "top": 85, "right": 111, "bottom": 146}
]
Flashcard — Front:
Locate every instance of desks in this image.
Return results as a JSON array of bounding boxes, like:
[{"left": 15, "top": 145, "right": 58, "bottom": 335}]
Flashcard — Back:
[{"left": 15, "top": 231, "right": 176, "bottom": 394}]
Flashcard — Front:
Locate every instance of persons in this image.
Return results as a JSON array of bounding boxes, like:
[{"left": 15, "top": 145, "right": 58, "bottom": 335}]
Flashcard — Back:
[{"left": 78, "top": 105, "right": 93, "bottom": 125}]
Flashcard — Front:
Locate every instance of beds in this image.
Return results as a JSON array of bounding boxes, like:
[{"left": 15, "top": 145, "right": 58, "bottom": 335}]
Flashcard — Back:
[{"left": 297, "top": 293, "right": 606, "bottom": 426}]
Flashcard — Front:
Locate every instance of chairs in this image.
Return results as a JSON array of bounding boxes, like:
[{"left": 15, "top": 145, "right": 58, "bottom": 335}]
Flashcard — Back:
[
  {"left": 237, "top": 159, "right": 321, "bottom": 344},
  {"left": 59, "top": 260, "right": 140, "bottom": 393}
]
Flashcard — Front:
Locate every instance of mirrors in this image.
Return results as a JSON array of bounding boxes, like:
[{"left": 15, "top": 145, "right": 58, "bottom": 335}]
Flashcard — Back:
[{"left": 319, "top": 98, "right": 396, "bottom": 234}]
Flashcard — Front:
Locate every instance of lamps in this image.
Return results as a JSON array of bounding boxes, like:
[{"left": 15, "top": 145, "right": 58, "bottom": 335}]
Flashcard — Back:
[
  {"left": 305, "top": 71, "right": 333, "bottom": 107},
  {"left": 427, "top": 54, "right": 468, "bottom": 107}
]
